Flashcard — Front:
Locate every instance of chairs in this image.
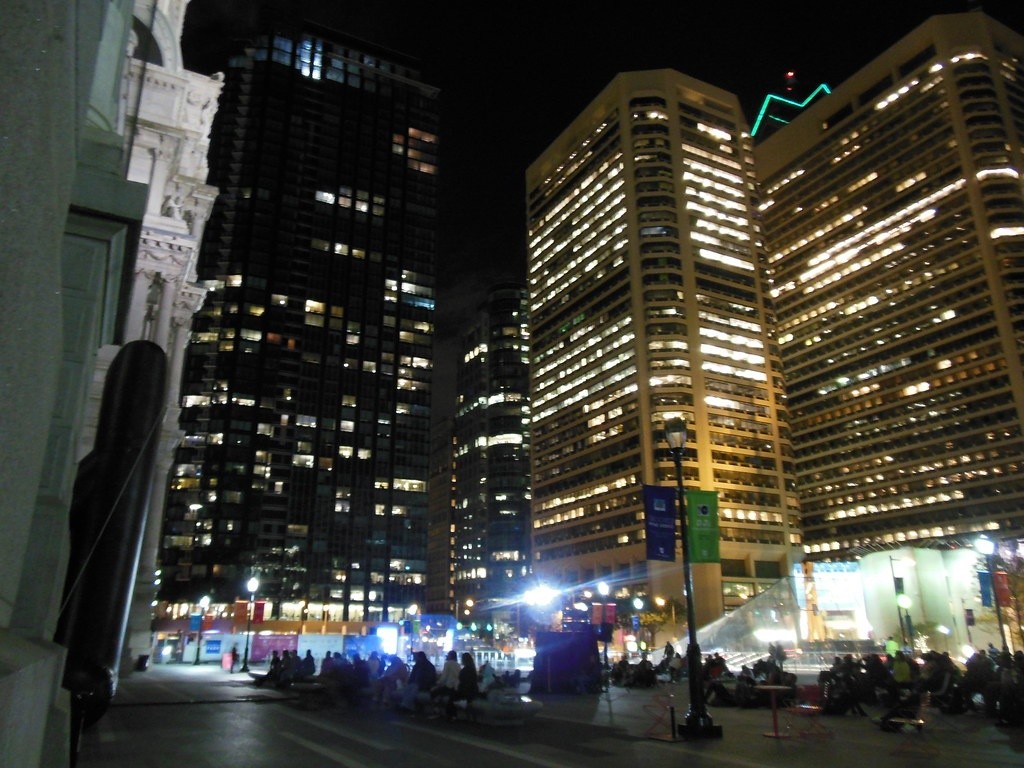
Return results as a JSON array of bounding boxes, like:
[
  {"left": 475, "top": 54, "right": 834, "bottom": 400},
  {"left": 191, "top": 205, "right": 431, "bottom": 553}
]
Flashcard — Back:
[
  {"left": 888, "top": 691, "right": 939, "bottom": 757},
  {"left": 781, "top": 683, "right": 831, "bottom": 737},
  {"left": 641, "top": 681, "right": 688, "bottom": 736}
]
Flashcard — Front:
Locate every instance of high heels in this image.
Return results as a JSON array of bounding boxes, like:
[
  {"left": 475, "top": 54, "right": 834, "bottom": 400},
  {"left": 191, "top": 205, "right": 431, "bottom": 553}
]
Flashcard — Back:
[{"left": 445, "top": 718, "right": 453, "bottom": 722}]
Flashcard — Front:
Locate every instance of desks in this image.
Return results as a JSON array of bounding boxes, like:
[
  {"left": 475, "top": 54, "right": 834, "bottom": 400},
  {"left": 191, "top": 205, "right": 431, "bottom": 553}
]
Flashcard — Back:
[{"left": 755, "top": 686, "right": 792, "bottom": 739}]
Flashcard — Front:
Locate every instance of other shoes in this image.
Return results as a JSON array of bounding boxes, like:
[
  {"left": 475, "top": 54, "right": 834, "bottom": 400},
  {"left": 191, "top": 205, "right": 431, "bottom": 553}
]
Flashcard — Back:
[
  {"left": 428, "top": 715, "right": 439, "bottom": 719},
  {"left": 406, "top": 714, "right": 414, "bottom": 718},
  {"left": 994, "top": 719, "right": 1008, "bottom": 725}
]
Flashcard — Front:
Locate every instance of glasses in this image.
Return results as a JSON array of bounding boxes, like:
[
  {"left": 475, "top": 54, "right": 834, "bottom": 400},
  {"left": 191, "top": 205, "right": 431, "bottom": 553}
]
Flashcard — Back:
[{"left": 284, "top": 653, "right": 287, "bottom": 654}]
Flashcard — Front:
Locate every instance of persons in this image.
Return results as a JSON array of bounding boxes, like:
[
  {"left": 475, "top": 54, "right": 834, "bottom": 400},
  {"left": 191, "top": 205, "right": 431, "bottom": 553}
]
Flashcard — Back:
[
  {"left": 253, "top": 647, "right": 478, "bottom": 722},
  {"left": 230, "top": 642, "right": 239, "bottom": 673},
  {"left": 479, "top": 642, "right": 1024, "bottom": 730}
]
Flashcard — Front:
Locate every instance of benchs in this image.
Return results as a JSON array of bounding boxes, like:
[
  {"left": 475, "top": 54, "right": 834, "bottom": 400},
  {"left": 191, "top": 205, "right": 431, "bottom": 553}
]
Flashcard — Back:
[{"left": 304, "top": 675, "right": 317, "bottom": 683}]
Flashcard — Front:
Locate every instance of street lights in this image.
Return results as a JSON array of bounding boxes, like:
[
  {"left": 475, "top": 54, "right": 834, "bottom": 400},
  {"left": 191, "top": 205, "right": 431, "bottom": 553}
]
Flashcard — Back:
[
  {"left": 887, "top": 553, "right": 917, "bottom": 653},
  {"left": 238, "top": 576, "right": 259, "bottom": 673},
  {"left": 597, "top": 581, "right": 610, "bottom": 667},
  {"left": 894, "top": 594, "right": 917, "bottom": 658},
  {"left": 192, "top": 595, "right": 210, "bottom": 665},
  {"left": 663, "top": 415, "right": 724, "bottom": 740},
  {"left": 407, "top": 604, "right": 419, "bottom": 663},
  {"left": 973, "top": 533, "right": 1009, "bottom": 649}
]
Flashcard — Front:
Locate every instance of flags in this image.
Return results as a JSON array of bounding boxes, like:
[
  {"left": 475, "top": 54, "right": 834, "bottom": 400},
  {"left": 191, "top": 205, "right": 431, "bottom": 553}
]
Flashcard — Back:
[
  {"left": 992, "top": 571, "right": 1011, "bottom": 608},
  {"left": 977, "top": 571, "right": 993, "bottom": 608}
]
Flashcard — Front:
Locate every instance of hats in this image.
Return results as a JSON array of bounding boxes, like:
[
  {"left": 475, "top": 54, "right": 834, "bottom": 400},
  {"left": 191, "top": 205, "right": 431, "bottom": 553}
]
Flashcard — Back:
[{"left": 290, "top": 650, "right": 297, "bottom": 653}]
